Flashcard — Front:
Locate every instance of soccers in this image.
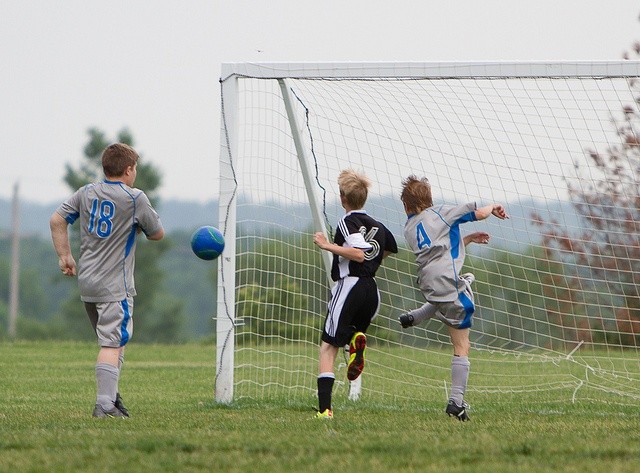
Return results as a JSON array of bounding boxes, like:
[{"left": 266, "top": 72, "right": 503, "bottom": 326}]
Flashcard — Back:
[{"left": 190, "top": 226, "right": 225, "bottom": 260}]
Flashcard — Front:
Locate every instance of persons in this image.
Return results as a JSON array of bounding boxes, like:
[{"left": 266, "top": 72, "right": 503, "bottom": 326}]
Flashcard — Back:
[
  {"left": 49, "top": 143, "right": 166, "bottom": 421},
  {"left": 398, "top": 174, "right": 511, "bottom": 421},
  {"left": 312, "top": 168, "right": 399, "bottom": 420}
]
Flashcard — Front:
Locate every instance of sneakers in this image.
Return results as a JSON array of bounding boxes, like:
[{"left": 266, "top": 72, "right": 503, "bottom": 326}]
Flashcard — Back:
[
  {"left": 398, "top": 313, "right": 414, "bottom": 328},
  {"left": 347, "top": 332, "right": 366, "bottom": 380},
  {"left": 446, "top": 399, "right": 470, "bottom": 421},
  {"left": 306, "top": 409, "right": 333, "bottom": 419},
  {"left": 114, "top": 393, "right": 129, "bottom": 417},
  {"left": 92, "top": 404, "right": 128, "bottom": 420}
]
